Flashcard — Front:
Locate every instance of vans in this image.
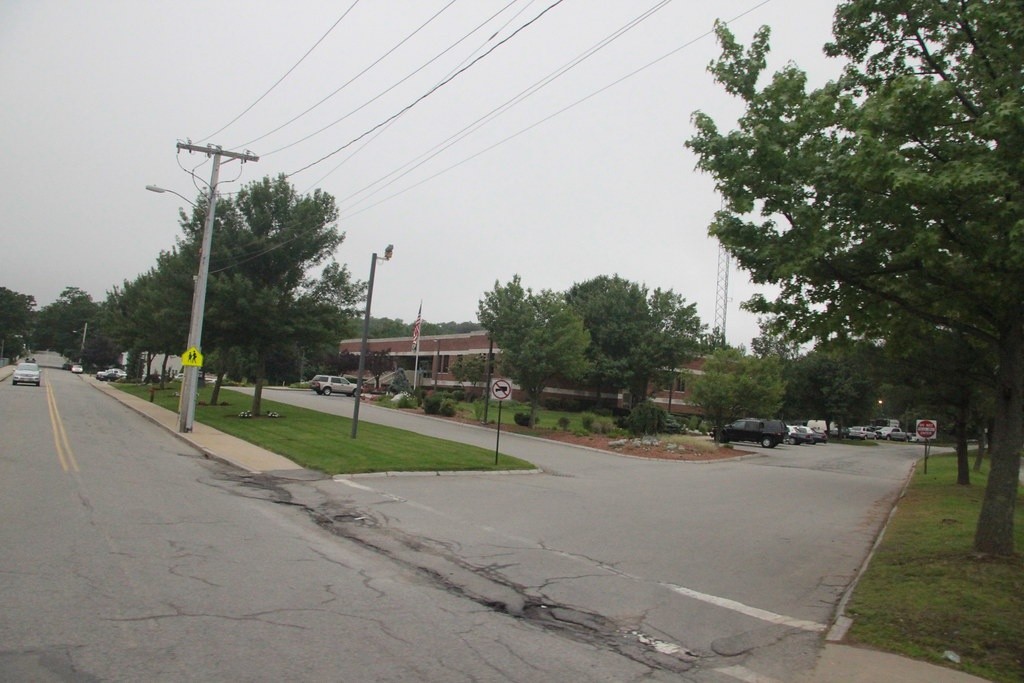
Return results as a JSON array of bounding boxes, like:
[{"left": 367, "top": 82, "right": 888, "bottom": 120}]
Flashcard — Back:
[{"left": 807, "top": 419, "right": 827, "bottom": 432}]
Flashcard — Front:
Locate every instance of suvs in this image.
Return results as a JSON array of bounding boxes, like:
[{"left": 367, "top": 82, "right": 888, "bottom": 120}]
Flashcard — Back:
[{"left": 310, "top": 374, "right": 364, "bottom": 397}]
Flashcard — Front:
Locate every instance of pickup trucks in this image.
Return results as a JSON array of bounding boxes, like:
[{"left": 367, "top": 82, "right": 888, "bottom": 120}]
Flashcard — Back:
[
  {"left": 876, "top": 426, "right": 912, "bottom": 442},
  {"left": 709, "top": 418, "right": 786, "bottom": 448}
]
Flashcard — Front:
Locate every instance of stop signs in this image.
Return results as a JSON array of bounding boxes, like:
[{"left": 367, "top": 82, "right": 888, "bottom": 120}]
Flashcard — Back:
[{"left": 916, "top": 419, "right": 937, "bottom": 440}]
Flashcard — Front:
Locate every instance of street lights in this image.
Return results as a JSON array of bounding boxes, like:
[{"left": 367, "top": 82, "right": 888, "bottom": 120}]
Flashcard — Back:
[
  {"left": 432, "top": 338, "right": 441, "bottom": 389},
  {"left": 146, "top": 184, "right": 215, "bottom": 433},
  {"left": 351, "top": 244, "right": 395, "bottom": 440}
]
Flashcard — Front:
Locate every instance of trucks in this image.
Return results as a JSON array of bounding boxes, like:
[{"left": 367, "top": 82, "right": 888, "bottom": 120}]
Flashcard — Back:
[{"left": 876, "top": 419, "right": 900, "bottom": 428}]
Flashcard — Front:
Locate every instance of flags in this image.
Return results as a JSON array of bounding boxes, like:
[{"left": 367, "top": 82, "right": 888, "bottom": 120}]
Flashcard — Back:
[{"left": 412, "top": 305, "right": 421, "bottom": 353}]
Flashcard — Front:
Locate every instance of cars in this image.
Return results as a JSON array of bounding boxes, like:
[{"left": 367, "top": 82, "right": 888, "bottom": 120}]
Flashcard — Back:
[
  {"left": 910, "top": 433, "right": 925, "bottom": 443},
  {"left": 12, "top": 362, "right": 43, "bottom": 386},
  {"left": 71, "top": 365, "right": 84, "bottom": 374},
  {"left": 63, "top": 364, "right": 71, "bottom": 371},
  {"left": 96, "top": 368, "right": 127, "bottom": 383},
  {"left": 849, "top": 425, "right": 878, "bottom": 440},
  {"left": 785, "top": 425, "right": 827, "bottom": 445},
  {"left": 828, "top": 422, "right": 849, "bottom": 439}
]
